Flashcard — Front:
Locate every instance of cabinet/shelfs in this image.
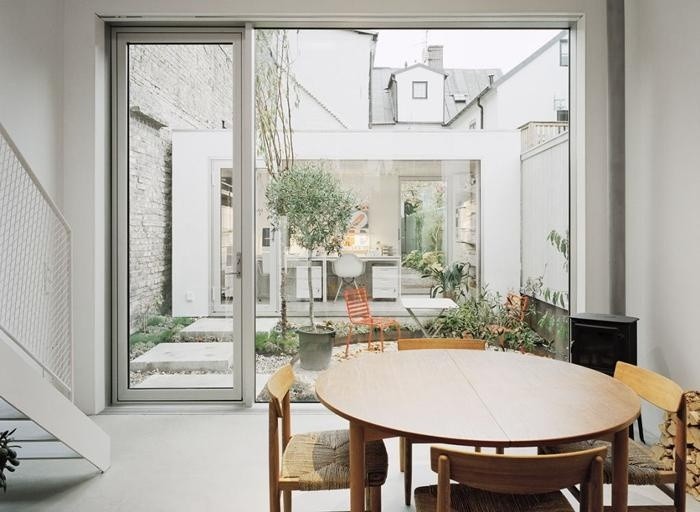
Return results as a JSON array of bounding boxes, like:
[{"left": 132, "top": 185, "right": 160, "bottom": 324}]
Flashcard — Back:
[
  {"left": 568, "top": 312, "right": 646, "bottom": 446},
  {"left": 372, "top": 266, "right": 398, "bottom": 299},
  {"left": 296, "top": 266, "right": 323, "bottom": 299}
]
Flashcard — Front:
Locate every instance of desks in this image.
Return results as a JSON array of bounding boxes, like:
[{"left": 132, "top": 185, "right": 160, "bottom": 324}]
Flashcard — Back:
[
  {"left": 311, "top": 257, "right": 401, "bottom": 302},
  {"left": 315, "top": 348, "right": 641, "bottom": 512}
]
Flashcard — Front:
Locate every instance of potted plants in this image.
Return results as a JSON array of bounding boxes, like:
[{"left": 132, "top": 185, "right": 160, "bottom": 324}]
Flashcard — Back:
[
  {"left": 421, "top": 262, "right": 472, "bottom": 303},
  {"left": 266, "top": 159, "right": 369, "bottom": 371}
]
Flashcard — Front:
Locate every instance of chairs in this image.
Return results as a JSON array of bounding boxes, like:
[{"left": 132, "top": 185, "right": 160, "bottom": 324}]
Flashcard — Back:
[
  {"left": 331, "top": 253, "right": 365, "bottom": 303},
  {"left": 462, "top": 293, "right": 528, "bottom": 348},
  {"left": 269, "top": 364, "right": 389, "bottom": 512},
  {"left": 397, "top": 339, "right": 505, "bottom": 505},
  {"left": 342, "top": 287, "right": 401, "bottom": 358},
  {"left": 414, "top": 445, "right": 608, "bottom": 512},
  {"left": 537, "top": 360, "right": 687, "bottom": 512}
]
